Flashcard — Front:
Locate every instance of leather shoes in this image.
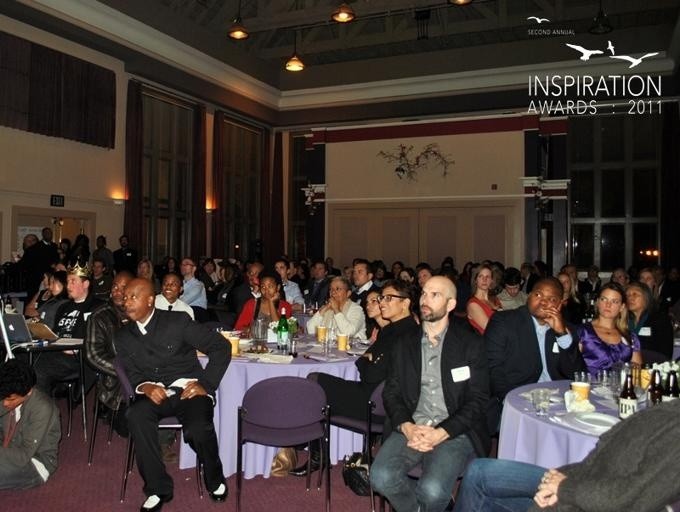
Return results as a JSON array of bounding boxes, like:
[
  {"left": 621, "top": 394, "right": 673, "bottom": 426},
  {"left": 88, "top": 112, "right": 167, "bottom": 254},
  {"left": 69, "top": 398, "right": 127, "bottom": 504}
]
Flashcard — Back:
[
  {"left": 288, "top": 460, "right": 331, "bottom": 476},
  {"left": 210, "top": 476, "right": 228, "bottom": 501},
  {"left": 141, "top": 493, "right": 173, "bottom": 511}
]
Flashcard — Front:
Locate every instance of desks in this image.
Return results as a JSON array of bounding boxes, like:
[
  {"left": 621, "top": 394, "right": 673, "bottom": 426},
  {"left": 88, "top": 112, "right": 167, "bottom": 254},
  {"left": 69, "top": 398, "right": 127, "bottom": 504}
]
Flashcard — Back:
[{"left": 13, "top": 344, "right": 88, "bottom": 443}]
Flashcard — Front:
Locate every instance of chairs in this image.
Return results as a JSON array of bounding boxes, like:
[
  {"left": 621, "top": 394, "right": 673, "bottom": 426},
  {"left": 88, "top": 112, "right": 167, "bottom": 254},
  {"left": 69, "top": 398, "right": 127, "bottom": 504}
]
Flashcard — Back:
[
  {"left": 236, "top": 377, "right": 331, "bottom": 511},
  {"left": 113, "top": 357, "right": 203, "bottom": 503},
  {"left": 88, "top": 388, "right": 134, "bottom": 476},
  {"left": 55, "top": 372, "right": 78, "bottom": 436},
  {"left": 318, "top": 380, "right": 388, "bottom": 512}
]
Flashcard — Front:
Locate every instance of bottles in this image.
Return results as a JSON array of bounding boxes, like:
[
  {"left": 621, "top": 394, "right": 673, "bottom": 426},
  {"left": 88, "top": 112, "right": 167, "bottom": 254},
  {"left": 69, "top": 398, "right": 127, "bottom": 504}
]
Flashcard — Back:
[
  {"left": 4, "top": 294, "right": 13, "bottom": 309},
  {"left": 574, "top": 369, "right": 619, "bottom": 387},
  {"left": 665, "top": 370, "right": 680, "bottom": 397},
  {"left": 312, "top": 301, "right": 319, "bottom": 314},
  {"left": 587, "top": 297, "right": 596, "bottom": 318},
  {"left": 640, "top": 363, "right": 652, "bottom": 390},
  {"left": 277, "top": 307, "right": 288, "bottom": 349},
  {"left": 619, "top": 368, "right": 637, "bottom": 420},
  {"left": 648, "top": 369, "right": 664, "bottom": 407},
  {"left": 298, "top": 304, "right": 309, "bottom": 343},
  {"left": 289, "top": 340, "right": 298, "bottom": 358}
]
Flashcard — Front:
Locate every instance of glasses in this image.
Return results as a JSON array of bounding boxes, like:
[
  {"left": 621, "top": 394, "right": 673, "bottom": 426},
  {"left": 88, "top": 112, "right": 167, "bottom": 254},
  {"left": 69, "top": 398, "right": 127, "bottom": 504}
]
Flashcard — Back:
[
  {"left": 179, "top": 262, "right": 194, "bottom": 267},
  {"left": 378, "top": 293, "right": 406, "bottom": 302}
]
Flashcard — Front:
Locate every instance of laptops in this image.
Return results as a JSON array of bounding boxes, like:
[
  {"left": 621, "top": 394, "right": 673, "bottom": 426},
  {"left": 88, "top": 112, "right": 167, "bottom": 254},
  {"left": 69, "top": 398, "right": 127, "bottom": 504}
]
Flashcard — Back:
[{"left": 0, "top": 313, "right": 47, "bottom": 342}]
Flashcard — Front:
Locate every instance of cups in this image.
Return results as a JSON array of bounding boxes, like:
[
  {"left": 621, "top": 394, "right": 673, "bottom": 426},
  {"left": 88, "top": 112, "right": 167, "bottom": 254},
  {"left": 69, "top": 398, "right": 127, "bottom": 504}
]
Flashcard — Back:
[
  {"left": 229, "top": 337, "right": 240, "bottom": 356},
  {"left": 571, "top": 382, "right": 592, "bottom": 401},
  {"left": 221, "top": 331, "right": 232, "bottom": 340},
  {"left": 337, "top": 334, "right": 348, "bottom": 351},
  {"left": 532, "top": 388, "right": 549, "bottom": 415},
  {"left": 317, "top": 326, "right": 326, "bottom": 342},
  {"left": 252, "top": 318, "right": 267, "bottom": 353}
]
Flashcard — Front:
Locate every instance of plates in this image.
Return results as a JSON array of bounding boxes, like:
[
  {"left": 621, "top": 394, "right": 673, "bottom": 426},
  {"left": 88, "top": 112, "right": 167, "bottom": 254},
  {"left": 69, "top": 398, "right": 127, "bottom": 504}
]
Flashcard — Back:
[{"left": 574, "top": 411, "right": 622, "bottom": 429}]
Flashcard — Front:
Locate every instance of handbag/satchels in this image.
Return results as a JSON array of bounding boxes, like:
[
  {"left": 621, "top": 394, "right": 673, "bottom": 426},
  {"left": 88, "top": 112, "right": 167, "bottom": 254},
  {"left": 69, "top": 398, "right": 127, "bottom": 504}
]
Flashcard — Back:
[{"left": 343, "top": 452, "right": 379, "bottom": 496}]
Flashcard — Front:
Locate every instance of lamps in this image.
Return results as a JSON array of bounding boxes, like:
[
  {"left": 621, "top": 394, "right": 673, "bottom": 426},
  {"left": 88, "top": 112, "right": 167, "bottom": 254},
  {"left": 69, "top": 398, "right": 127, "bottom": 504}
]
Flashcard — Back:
[
  {"left": 333, "top": 0, "right": 353, "bottom": 21},
  {"left": 112, "top": 191, "right": 127, "bottom": 205},
  {"left": 230, "top": 3, "right": 249, "bottom": 39},
  {"left": 286, "top": 27, "right": 303, "bottom": 71}
]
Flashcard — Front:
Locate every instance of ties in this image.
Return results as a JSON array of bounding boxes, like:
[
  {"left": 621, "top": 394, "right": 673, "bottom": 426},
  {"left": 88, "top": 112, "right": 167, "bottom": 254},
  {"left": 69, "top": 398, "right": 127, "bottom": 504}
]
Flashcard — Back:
[{"left": 167, "top": 305, "right": 173, "bottom": 311}]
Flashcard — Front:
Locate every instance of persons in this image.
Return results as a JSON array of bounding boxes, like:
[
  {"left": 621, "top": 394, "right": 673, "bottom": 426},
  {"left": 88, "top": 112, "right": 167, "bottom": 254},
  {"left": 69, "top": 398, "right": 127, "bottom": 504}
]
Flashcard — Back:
[{"left": 0, "top": 227, "right": 680, "bottom": 512}]
